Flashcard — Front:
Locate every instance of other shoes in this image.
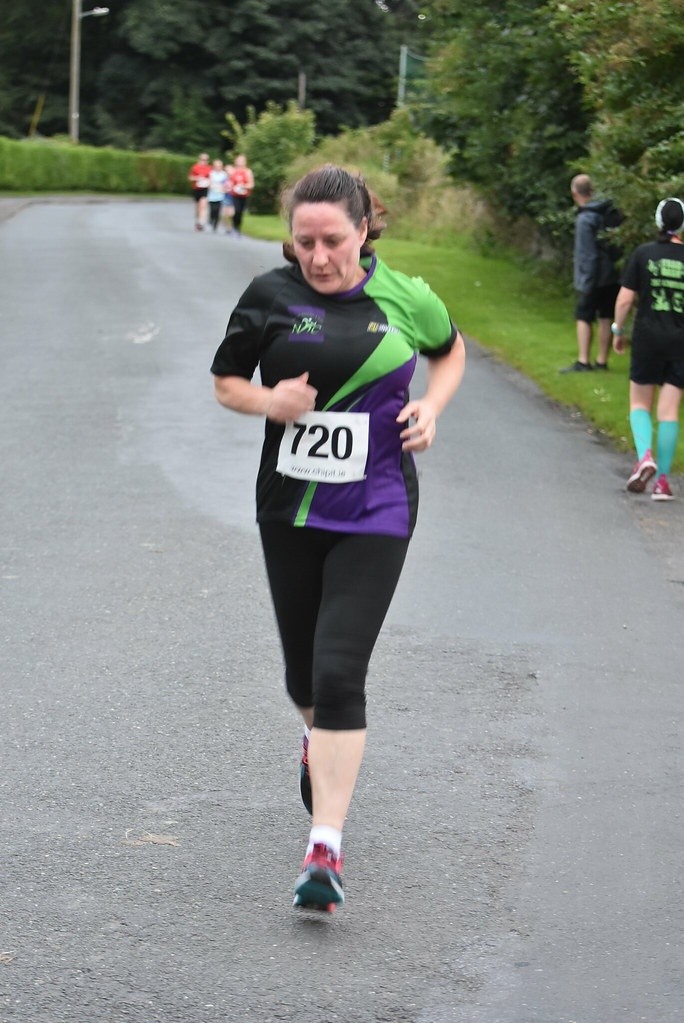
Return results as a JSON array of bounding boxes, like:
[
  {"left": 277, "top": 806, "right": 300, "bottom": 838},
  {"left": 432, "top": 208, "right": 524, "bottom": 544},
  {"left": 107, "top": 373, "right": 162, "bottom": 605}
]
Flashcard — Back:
[
  {"left": 561, "top": 363, "right": 610, "bottom": 374},
  {"left": 195, "top": 221, "right": 242, "bottom": 239}
]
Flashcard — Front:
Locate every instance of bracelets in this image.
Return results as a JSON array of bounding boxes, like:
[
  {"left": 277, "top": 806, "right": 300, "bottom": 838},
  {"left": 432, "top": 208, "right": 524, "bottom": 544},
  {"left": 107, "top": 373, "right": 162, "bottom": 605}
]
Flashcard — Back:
[{"left": 611, "top": 321, "right": 625, "bottom": 336}]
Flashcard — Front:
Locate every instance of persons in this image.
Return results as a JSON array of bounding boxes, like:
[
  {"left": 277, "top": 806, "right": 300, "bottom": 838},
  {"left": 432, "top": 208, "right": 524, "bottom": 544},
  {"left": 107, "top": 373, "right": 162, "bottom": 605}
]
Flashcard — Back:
[
  {"left": 188, "top": 153, "right": 255, "bottom": 238},
  {"left": 610, "top": 197, "right": 684, "bottom": 501},
  {"left": 559, "top": 173, "right": 619, "bottom": 374},
  {"left": 211, "top": 165, "right": 465, "bottom": 923}
]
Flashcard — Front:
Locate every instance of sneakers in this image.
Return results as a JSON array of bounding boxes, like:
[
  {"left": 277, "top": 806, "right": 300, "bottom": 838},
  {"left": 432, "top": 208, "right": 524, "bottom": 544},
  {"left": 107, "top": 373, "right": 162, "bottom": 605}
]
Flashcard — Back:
[
  {"left": 294, "top": 841, "right": 348, "bottom": 913},
  {"left": 652, "top": 475, "right": 677, "bottom": 502},
  {"left": 298, "top": 734, "right": 319, "bottom": 815},
  {"left": 625, "top": 451, "right": 658, "bottom": 494}
]
